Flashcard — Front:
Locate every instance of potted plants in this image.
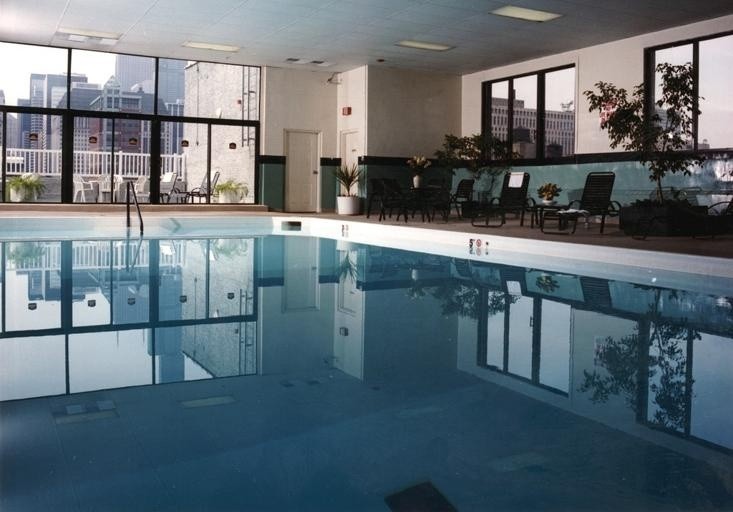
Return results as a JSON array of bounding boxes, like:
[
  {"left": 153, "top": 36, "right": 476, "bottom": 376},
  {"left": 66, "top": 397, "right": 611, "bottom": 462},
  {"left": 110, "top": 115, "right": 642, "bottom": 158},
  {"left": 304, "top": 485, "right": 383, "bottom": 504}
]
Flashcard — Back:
[
  {"left": 6, "top": 242, "right": 45, "bottom": 263},
  {"left": 444, "top": 129, "right": 525, "bottom": 203},
  {"left": 583, "top": 62, "right": 709, "bottom": 235},
  {"left": 215, "top": 237, "right": 248, "bottom": 257},
  {"left": 536, "top": 271, "right": 560, "bottom": 293},
  {"left": 405, "top": 267, "right": 426, "bottom": 300},
  {"left": 7, "top": 172, "right": 45, "bottom": 202},
  {"left": 215, "top": 180, "right": 249, "bottom": 203},
  {"left": 332, "top": 163, "right": 365, "bottom": 216},
  {"left": 331, "top": 240, "right": 362, "bottom": 284}
]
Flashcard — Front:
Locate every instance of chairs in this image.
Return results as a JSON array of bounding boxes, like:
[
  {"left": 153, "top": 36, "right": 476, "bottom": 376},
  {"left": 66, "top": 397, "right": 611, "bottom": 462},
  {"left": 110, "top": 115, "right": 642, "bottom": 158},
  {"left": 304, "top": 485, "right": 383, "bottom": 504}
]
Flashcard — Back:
[
  {"left": 367, "top": 246, "right": 493, "bottom": 278},
  {"left": 69, "top": 238, "right": 220, "bottom": 261},
  {"left": 71, "top": 171, "right": 221, "bottom": 204},
  {"left": 579, "top": 277, "right": 613, "bottom": 309},
  {"left": 476, "top": 262, "right": 527, "bottom": 296}
]
